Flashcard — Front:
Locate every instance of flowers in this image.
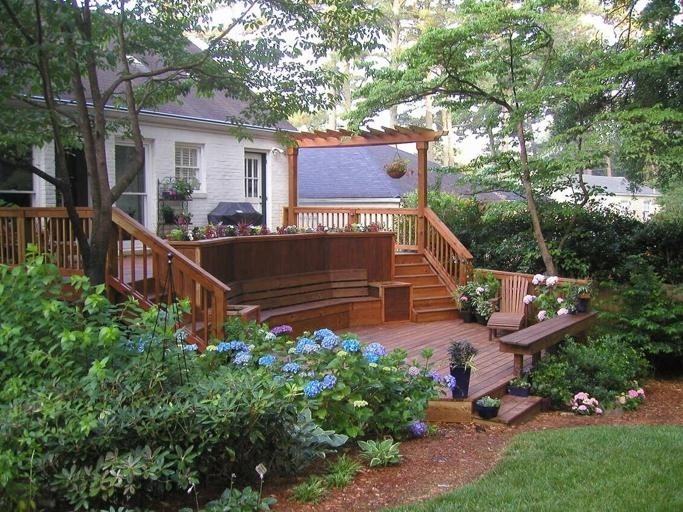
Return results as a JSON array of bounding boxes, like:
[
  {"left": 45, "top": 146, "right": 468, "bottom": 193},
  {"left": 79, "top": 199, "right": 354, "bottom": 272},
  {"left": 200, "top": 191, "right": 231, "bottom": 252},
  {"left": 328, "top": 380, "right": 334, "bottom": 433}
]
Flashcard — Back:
[{"left": 448, "top": 281, "right": 498, "bottom": 320}]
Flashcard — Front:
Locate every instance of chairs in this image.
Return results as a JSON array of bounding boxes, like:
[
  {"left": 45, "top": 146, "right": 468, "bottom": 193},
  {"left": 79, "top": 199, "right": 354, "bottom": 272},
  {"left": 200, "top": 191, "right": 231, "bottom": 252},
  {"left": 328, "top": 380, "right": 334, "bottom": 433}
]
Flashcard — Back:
[{"left": 486, "top": 275, "right": 528, "bottom": 342}]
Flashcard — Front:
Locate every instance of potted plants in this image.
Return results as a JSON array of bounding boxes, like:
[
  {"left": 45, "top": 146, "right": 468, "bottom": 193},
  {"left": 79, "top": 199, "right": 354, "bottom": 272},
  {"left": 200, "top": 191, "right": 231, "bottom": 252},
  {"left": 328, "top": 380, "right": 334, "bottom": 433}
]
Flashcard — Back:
[
  {"left": 161, "top": 179, "right": 193, "bottom": 225},
  {"left": 574, "top": 284, "right": 592, "bottom": 313},
  {"left": 506, "top": 368, "right": 532, "bottom": 396},
  {"left": 446, "top": 335, "right": 480, "bottom": 399},
  {"left": 475, "top": 394, "right": 500, "bottom": 419},
  {"left": 383, "top": 159, "right": 411, "bottom": 178}
]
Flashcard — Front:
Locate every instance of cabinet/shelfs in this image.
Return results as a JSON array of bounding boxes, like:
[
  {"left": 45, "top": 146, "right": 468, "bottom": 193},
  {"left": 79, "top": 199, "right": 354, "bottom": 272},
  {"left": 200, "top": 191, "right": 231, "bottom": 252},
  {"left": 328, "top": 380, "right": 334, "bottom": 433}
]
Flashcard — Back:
[{"left": 156, "top": 176, "right": 193, "bottom": 238}]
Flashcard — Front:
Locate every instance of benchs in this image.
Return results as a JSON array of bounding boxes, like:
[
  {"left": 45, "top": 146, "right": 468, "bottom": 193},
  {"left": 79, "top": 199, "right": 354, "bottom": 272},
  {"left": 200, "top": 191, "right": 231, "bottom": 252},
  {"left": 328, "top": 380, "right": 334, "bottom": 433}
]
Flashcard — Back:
[{"left": 496, "top": 310, "right": 599, "bottom": 380}]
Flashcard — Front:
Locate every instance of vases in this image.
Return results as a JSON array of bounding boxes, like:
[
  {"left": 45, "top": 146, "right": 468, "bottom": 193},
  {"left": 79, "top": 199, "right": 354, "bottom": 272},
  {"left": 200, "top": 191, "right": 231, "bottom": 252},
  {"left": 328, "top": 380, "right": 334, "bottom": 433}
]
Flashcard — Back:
[{"left": 459, "top": 308, "right": 486, "bottom": 324}]
